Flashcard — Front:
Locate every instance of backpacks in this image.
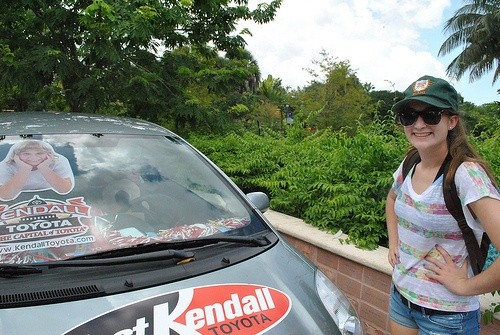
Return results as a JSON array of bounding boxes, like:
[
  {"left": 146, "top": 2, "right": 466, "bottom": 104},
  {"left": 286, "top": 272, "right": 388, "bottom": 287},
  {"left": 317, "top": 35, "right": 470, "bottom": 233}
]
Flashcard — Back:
[{"left": 402, "top": 147, "right": 491, "bottom": 277}]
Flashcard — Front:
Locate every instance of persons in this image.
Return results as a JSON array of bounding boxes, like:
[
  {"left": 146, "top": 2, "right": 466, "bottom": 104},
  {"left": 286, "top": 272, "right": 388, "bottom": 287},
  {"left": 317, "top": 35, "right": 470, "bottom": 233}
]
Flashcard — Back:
[
  {"left": 0, "top": 140, "right": 75, "bottom": 200},
  {"left": 385, "top": 75, "right": 500, "bottom": 335}
]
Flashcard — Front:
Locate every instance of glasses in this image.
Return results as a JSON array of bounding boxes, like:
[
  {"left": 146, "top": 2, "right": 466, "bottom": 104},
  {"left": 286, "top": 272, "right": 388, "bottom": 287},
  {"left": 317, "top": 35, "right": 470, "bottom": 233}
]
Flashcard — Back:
[{"left": 398, "top": 106, "right": 449, "bottom": 126}]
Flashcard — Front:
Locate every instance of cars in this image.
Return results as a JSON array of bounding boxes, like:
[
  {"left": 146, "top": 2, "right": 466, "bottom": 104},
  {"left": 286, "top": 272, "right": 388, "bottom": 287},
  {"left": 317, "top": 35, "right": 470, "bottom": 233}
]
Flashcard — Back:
[{"left": 0, "top": 113, "right": 367, "bottom": 335}]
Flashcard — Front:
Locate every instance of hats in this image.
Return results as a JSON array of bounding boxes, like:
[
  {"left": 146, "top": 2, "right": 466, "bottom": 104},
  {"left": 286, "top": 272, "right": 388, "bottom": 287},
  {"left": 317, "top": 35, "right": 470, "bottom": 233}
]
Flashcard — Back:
[{"left": 392, "top": 75, "right": 458, "bottom": 113}]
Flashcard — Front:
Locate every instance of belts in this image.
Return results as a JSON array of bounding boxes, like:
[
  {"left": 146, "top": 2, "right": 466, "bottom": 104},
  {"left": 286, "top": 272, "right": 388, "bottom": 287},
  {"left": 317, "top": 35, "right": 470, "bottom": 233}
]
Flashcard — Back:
[{"left": 394, "top": 285, "right": 466, "bottom": 315}]
